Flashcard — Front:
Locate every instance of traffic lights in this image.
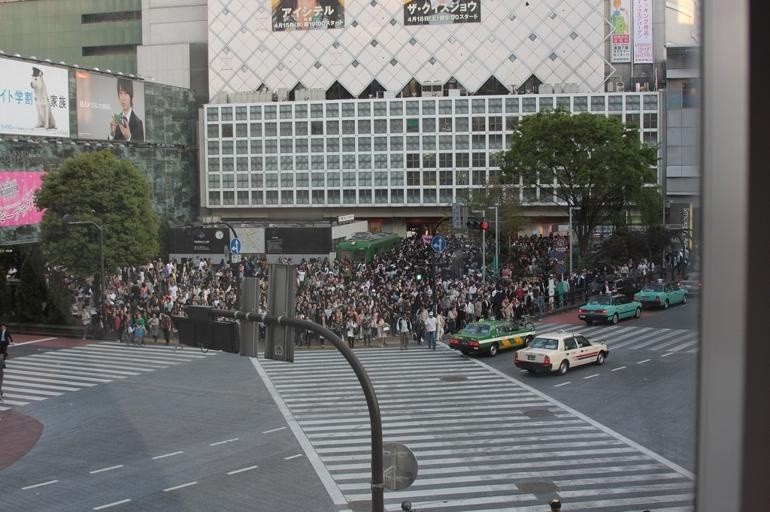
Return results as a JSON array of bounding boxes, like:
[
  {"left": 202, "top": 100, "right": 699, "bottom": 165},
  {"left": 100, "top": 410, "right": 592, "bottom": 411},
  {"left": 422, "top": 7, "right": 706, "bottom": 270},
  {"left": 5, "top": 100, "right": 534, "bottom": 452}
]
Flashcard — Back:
[{"left": 466, "top": 219, "right": 489, "bottom": 230}]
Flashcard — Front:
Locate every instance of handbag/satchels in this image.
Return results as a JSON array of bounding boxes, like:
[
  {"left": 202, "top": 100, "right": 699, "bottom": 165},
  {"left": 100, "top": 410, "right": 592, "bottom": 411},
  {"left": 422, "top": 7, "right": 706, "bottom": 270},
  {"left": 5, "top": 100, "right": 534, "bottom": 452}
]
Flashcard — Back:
[
  {"left": 353, "top": 325, "right": 360, "bottom": 334},
  {"left": 382, "top": 327, "right": 390, "bottom": 332}
]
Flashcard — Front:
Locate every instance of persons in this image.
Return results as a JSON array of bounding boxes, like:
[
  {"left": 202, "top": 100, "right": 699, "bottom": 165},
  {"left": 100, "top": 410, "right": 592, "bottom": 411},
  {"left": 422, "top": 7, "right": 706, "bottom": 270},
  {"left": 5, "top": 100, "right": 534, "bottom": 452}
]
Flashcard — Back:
[
  {"left": 0, "top": 353, "right": 8, "bottom": 403},
  {"left": 0, "top": 228, "right": 692, "bottom": 353},
  {"left": 271, "top": 0, "right": 345, "bottom": 30},
  {"left": 107, "top": 77, "right": 144, "bottom": 143},
  {"left": 0, "top": 323, "right": 14, "bottom": 361}
]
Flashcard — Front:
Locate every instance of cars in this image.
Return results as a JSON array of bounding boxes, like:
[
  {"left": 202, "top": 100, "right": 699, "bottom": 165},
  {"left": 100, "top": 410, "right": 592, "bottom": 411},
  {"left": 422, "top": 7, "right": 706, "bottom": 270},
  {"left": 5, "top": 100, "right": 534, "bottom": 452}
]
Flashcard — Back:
[
  {"left": 633, "top": 278, "right": 689, "bottom": 310},
  {"left": 676, "top": 271, "right": 702, "bottom": 296},
  {"left": 513, "top": 328, "right": 610, "bottom": 376},
  {"left": 449, "top": 319, "right": 537, "bottom": 358},
  {"left": 578, "top": 289, "right": 644, "bottom": 325}
]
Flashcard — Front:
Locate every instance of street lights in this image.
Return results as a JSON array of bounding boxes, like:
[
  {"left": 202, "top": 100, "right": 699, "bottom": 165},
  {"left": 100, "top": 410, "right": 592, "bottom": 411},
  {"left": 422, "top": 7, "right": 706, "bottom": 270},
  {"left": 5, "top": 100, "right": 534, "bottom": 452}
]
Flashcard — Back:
[
  {"left": 569, "top": 206, "right": 581, "bottom": 276},
  {"left": 487, "top": 206, "right": 499, "bottom": 272}
]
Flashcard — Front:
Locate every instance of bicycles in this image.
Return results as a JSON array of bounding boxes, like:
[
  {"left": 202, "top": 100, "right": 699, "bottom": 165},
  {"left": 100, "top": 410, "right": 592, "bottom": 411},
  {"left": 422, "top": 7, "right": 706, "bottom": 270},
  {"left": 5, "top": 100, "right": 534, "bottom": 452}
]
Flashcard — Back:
[{"left": 514, "top": 315, "right": 537, "bottom": 330}]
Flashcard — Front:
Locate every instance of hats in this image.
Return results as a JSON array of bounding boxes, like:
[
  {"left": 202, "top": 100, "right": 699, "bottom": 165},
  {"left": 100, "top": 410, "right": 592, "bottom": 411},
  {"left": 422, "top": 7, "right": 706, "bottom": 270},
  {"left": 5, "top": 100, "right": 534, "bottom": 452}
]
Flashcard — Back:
[{"left": 429, "top": 312, "right": 433, "bottom": 316}]
